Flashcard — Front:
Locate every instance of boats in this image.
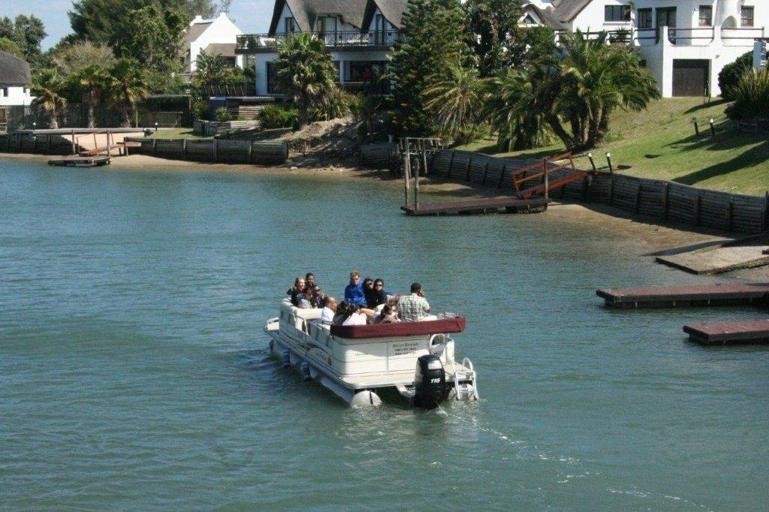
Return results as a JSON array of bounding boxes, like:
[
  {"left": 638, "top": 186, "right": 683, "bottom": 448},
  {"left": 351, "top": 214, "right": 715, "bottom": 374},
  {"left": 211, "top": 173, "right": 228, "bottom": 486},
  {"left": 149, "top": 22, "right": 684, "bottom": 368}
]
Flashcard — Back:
[{"left": 263, "top": 297, "right": 479, "bottom": 409}]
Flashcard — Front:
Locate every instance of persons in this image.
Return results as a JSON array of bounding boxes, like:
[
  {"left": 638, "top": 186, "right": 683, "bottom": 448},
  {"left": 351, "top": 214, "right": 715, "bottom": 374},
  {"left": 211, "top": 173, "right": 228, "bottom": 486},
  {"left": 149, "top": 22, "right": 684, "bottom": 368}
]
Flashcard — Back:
[{"left": 287, "top": 271, "right": 432, "bottom": 326}]
